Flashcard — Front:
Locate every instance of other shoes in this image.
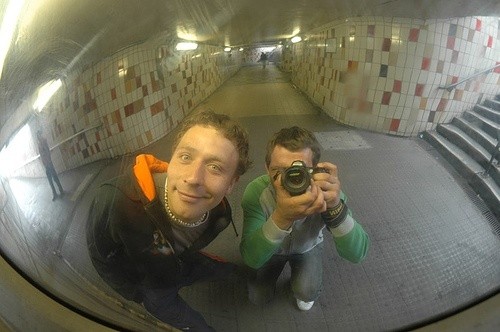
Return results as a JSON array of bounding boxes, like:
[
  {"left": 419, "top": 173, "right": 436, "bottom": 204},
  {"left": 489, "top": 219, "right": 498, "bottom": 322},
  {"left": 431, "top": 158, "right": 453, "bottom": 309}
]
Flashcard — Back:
[
  {"left": 296, "top": 299, "right": 316, "bottom": 311},
  {"left": 238, "top": 265, "right": 257, "bottom": 284},
  {"left": 51, "top": 192, "right": 66, "bottom": 201}
]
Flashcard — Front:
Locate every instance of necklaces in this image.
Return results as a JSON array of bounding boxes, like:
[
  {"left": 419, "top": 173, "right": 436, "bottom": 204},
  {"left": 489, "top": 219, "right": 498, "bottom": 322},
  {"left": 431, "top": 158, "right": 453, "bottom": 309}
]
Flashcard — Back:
[{"left": 164, "top": 181, "right": 207, "bottom": 226}]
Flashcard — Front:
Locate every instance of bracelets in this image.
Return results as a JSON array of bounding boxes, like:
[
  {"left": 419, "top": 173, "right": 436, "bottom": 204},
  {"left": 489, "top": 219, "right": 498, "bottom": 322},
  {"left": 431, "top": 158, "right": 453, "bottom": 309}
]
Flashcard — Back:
[{"left": 322, "top": 199, "right": 350, "bottom": 228}]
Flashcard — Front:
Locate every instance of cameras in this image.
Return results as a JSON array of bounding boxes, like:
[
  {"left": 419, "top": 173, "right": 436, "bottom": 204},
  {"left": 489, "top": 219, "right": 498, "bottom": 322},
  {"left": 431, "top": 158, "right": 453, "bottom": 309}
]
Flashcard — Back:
[{"left": 272, "top": 160, "right": 328, "bottom": 194}]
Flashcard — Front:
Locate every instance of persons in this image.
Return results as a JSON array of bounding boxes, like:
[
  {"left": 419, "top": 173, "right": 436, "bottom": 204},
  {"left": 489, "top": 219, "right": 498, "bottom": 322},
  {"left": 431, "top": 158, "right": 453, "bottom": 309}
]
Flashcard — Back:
[
  {"left": 85, "top": 109, "right": 252, "bottom": 332},
  {"left": 239, "top": 126, "right": 369, "bottom": 311},
  {"left": 261, "top": 53, "right": 268, "bottom": 69}
]
